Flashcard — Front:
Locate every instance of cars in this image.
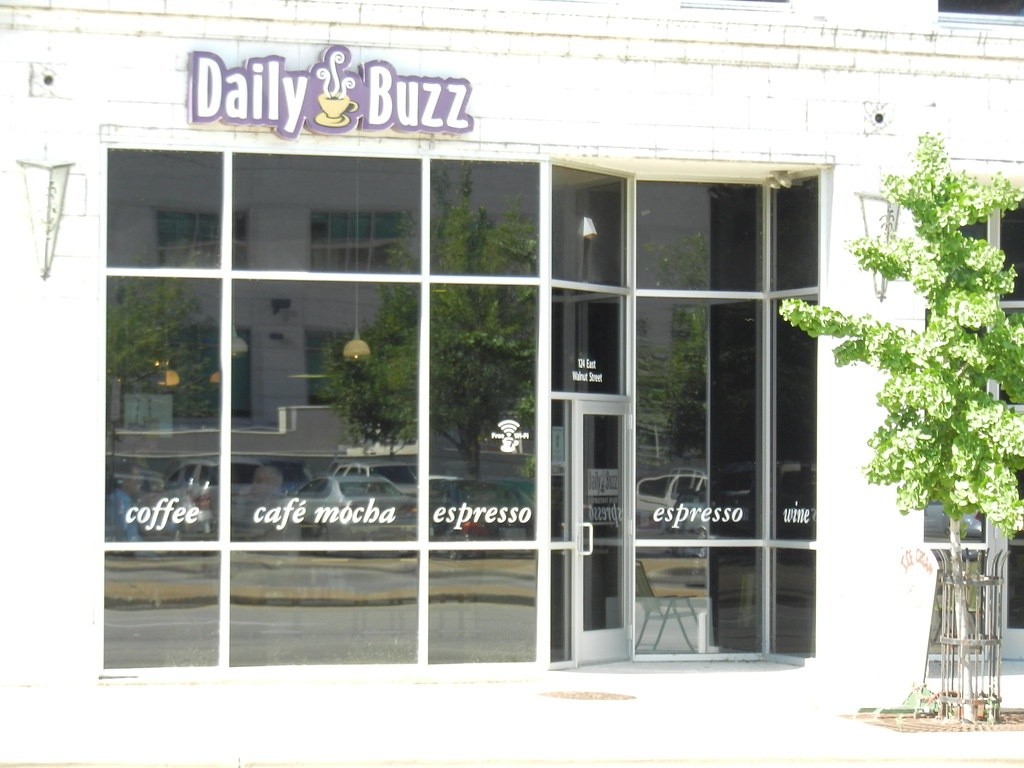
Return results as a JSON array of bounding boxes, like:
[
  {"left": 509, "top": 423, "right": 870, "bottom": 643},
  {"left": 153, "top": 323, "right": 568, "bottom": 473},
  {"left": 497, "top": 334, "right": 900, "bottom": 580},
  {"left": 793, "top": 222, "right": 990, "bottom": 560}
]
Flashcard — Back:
[
  {"left": 634, "top": 461, "right": 794, "bottom": 558},
  {"left": 267, "top": 460, "right": 418, "bottom": 496},
  {"left": 137, "top": 457, "right": 285, "bottom": 541},
  {"left": 114, "top": 476, "right": 163, "bottom": 501},
  {"left": 277, "top": 476, "right": 418, "bottom": 556},
  {"left": 429, "top": 476, "right": 537, "bottom": 558}
]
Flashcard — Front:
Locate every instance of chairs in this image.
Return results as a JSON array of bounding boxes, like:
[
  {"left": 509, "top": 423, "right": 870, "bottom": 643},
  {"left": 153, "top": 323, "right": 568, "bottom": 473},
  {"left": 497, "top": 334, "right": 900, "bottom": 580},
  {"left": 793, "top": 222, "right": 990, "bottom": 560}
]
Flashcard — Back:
[{"left": 633, "top": 560, "right": 700, "bottom": 654}]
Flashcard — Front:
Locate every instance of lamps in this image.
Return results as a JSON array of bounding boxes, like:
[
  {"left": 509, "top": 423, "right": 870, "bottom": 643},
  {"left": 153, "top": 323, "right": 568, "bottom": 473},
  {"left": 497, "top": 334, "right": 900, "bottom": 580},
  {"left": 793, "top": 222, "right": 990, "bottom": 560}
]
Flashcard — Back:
[
  {"left": 232, "top": 317, "right": 248, "bottom": 357},
  {"left": 767, "top": 171, "right": 792, "bottom": 190},
  {"left": 156, "top": 314, "right": 180, "bottom": 386},
  {"left": 341, "top": 156, "right": 372, "bottom": 361}
]
